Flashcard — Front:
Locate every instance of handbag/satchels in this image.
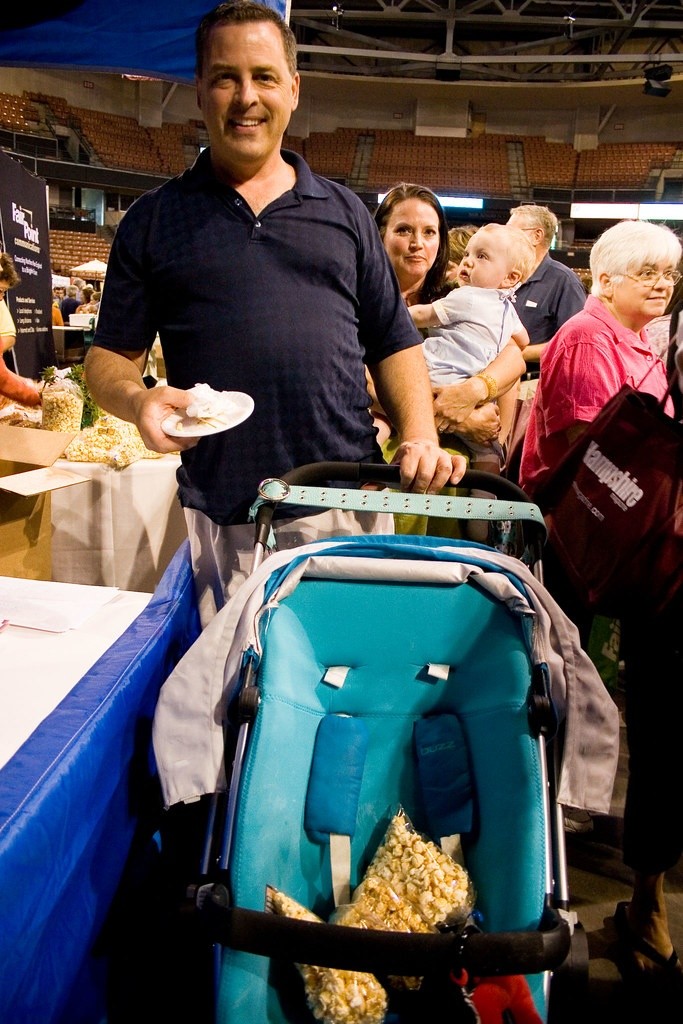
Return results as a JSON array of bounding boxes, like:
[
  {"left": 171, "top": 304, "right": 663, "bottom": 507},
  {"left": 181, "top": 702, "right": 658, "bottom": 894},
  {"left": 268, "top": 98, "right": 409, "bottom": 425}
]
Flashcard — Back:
[{"left": 532, "top": 336, "right": 683, "bottom": 619}]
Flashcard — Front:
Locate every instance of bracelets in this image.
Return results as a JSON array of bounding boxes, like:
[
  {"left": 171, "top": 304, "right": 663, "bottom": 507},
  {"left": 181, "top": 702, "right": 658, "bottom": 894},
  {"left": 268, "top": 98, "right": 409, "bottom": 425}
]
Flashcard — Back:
[{"left": 473, "top": 373, "right": 497, "bottom": 407}]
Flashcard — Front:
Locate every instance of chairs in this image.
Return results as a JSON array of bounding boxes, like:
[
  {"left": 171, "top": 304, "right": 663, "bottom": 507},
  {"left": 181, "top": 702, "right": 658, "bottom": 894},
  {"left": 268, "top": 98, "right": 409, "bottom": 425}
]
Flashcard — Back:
[
  {"left": 47, "top": 229, "right": 120, "bottom": 276},
  {"left": 0, "top": 91, "right": 683, "bottom": 200}
]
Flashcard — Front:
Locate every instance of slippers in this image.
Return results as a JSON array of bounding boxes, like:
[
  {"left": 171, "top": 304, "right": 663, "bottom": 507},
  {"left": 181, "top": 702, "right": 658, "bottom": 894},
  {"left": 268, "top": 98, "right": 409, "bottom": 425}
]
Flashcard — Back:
[{"left": 614, "top": 902, "right": 683, "bottom": 988}]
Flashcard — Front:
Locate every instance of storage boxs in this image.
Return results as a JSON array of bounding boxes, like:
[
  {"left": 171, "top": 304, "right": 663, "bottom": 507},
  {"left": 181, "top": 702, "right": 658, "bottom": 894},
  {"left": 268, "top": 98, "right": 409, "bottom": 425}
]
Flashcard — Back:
[{"left": 0, "top": 425, "right": 91, "bottom": 581}]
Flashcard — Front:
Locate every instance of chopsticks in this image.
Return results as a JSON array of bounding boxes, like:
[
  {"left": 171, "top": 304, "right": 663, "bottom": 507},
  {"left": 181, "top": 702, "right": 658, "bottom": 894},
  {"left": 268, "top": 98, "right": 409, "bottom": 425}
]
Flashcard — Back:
[{"left": 195, "top": 416, "right": 227, "bottom": 429}]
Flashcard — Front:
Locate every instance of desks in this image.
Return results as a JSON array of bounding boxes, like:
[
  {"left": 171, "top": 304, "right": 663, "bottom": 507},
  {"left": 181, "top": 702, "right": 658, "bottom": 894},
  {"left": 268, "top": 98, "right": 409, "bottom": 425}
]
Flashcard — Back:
[{"left": 51, "top": 376, "right": 189, "bottom": 594}]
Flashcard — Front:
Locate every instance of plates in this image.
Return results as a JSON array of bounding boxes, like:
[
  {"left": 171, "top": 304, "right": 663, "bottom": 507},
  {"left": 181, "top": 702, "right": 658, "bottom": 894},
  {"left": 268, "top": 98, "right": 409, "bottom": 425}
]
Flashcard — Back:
[{"left": 161, "top": 391, "right": 255, "bottom": 437}]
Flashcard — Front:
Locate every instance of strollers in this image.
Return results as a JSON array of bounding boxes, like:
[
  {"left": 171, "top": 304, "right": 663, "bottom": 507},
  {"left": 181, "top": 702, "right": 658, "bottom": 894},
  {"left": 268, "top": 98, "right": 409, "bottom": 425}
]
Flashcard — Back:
[{"left": 151, "top": 460, "right": 621, "bottom": 1024}]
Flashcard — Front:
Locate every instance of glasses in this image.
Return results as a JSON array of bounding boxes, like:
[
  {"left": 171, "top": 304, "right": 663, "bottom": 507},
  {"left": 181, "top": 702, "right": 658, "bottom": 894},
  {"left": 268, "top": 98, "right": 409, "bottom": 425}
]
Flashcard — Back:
[{"left": 628, "top": 271, "right": 682, "bottom": 287}]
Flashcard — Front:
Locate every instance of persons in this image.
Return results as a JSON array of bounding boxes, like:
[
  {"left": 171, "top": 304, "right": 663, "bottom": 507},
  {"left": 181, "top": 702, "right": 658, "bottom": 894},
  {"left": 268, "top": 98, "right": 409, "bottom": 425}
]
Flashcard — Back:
[
  {"left": 81, "top": 3, "right": 470, "bottom": 627},
  {"left": 0, "top": 179, "right": 683, "bottom": 539},
  {"left": 517, "top": 219, "right": 683, "bottom": 880},
  {"left": 552, "top": 312, "right": 683, "bottom": 1024}
]
